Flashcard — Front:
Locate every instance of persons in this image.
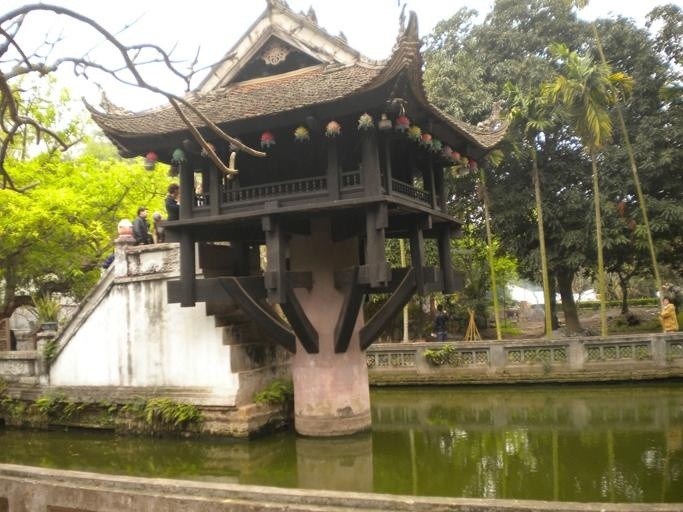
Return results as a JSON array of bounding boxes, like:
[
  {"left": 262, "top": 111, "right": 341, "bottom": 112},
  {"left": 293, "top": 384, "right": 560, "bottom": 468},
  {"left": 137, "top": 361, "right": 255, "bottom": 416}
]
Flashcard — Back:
[
  {"left": 431, "top": 304, "right": 449, "bottom": 342},
  {"left": 660, "top": 296, "right": 679, "bottom": 334},
  {"left": 101, "top": 184, "right": 203, "bottom": 269}
]
